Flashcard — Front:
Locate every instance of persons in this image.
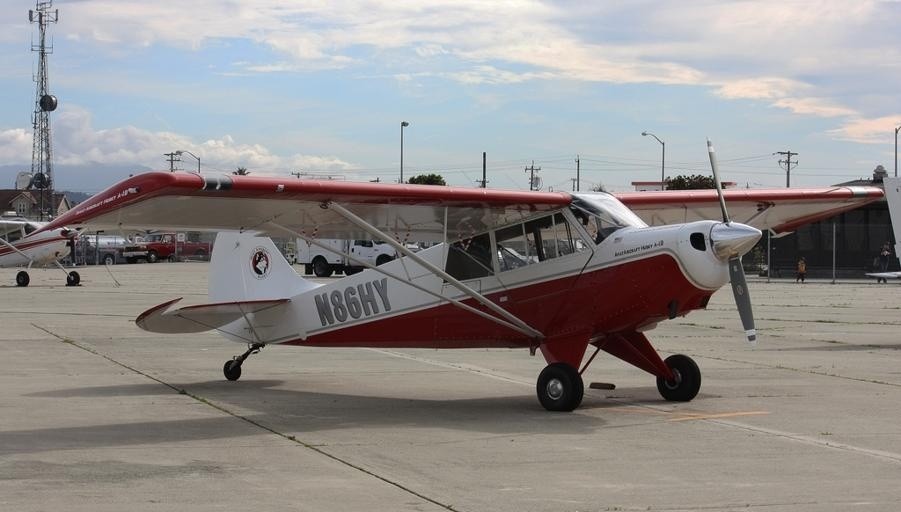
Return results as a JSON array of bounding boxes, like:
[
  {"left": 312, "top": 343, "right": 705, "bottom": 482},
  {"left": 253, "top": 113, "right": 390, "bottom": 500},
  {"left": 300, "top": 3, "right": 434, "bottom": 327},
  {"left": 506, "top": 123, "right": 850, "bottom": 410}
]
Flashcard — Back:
[
  {"left": 796, "top": 257, "right": 806, "bottom": 283},
  {"left": 877, "top": 241, "right": 890, "bottom": 283}
]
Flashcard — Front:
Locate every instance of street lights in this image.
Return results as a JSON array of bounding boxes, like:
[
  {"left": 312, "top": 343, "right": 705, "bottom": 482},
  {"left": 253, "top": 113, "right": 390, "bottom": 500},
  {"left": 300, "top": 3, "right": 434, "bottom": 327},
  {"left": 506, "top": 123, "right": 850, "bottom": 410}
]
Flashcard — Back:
[
  {"left": 399, "top": 119, "right": 409, "bottom": 183},
  {"left": 641, "top": 132, "right": 665, "bottom": 190},
  {"left": 175, "top": 148, "right": 200, "bottom": 173}
]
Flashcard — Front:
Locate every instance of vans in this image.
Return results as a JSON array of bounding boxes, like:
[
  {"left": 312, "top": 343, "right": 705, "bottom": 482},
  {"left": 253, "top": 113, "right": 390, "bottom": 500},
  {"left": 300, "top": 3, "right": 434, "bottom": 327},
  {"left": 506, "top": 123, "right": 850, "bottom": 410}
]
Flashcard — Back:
[{"left": 72, "top": 234, "right": 134, "bottom": 264}]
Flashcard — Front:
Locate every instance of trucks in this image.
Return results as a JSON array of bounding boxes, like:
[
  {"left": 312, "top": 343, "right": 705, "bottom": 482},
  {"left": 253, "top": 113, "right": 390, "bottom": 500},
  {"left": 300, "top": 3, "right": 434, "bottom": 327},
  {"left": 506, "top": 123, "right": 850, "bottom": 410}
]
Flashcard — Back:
[{"left": 290, "top": 235, "right": 400, "bottom": 278}]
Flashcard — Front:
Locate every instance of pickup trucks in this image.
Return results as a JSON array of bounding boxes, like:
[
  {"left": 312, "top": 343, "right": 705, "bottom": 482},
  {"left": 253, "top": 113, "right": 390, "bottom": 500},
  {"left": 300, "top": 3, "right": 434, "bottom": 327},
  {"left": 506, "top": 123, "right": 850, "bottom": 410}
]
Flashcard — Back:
[{"left": 123, "top": 232, "right": 211, "bottom": 263}]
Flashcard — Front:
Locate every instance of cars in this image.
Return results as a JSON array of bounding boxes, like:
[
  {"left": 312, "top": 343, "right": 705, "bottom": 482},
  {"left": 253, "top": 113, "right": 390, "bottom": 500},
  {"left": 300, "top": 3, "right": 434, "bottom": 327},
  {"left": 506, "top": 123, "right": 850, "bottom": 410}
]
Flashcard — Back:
[
  {"left": 404, "top": 241, "right": 423, "bottom": 252},
  {"left": 758, "top": 264, "right": 767, "bottom": 277}
]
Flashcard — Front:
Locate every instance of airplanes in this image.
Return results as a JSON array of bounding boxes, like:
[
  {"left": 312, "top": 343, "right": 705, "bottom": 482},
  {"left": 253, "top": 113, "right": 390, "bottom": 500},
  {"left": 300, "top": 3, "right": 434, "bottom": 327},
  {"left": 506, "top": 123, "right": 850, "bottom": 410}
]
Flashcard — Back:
[
  {"left": 19, "top": 136, "right": 888, "bottom": 411},
  {"left": 863, "top": 176, "right": 901, "bottom": 278},
  {"left": 0, "top": 219, "right": 82, "bottom": 289}
]
